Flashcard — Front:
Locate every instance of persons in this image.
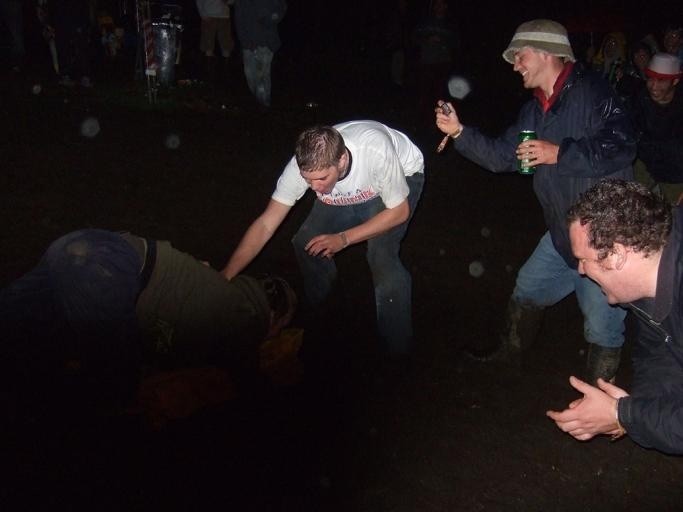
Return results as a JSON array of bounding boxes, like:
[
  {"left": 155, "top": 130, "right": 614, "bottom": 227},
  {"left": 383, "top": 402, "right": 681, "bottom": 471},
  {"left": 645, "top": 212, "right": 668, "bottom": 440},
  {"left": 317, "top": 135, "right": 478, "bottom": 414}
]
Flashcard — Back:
[
  {"left": 0, "top": 230, "right": 298, "bottom": 460},
  {"left": 413, "top": 0, "right": 458, "bottom": 140},
  {"left": 435, "top": 21, "right": 640, "bottom": 396},
  {"left": 546, "top": 175, "right": 683, "bottom": 457},
  {"left": 196, "top": 0, "right": 235, "bottom": 70},
  {"left": 219, "top": 119, "right": 425, "bottom": 345},
  {"left": 571, "top": 18, "right": 683, "bottom": 208},
  {"left": 233, "top": 1, "right": 288, "bottom": 113}
]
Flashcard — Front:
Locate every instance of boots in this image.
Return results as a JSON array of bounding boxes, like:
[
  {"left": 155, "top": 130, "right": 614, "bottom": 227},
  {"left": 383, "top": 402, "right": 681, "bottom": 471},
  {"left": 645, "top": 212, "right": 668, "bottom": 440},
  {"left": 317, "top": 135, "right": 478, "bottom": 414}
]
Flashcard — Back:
[
  {"left": 574, "top": 340, "right": 623, "bottom": 391},
  {"left": 466, "top": 295, "right": 547, "bottom": 371}
]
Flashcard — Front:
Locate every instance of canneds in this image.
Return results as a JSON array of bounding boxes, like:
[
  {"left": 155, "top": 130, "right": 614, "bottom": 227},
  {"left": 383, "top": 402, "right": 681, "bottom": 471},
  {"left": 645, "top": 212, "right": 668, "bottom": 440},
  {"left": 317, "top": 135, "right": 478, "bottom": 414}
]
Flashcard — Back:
[{"left": 518, "top": 130, "right": 537, "bottom": 175}]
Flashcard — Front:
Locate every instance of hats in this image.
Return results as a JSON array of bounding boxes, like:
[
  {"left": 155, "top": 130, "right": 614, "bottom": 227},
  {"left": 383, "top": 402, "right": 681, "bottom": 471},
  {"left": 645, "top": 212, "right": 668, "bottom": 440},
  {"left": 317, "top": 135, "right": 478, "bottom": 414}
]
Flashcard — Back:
[
  {"left": 501, "top": 17, "right": 578, "bottom": 66},
  {"left": 642, "top": 52, "right": 681, "bottom": 81}
]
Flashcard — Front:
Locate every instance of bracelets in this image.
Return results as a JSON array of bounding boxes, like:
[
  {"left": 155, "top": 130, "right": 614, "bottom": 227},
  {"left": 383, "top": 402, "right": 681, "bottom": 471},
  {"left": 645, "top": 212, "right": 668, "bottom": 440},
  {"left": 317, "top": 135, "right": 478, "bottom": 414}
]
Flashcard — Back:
[
  {"left": 339, "top": 231, "right": 350, "bottom": 248},
  {"left": 435, "top": 123, "right": 462, "bottom": 153}
]
